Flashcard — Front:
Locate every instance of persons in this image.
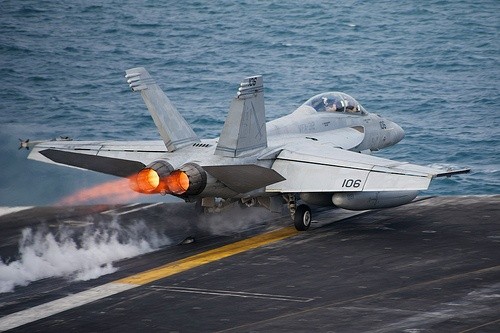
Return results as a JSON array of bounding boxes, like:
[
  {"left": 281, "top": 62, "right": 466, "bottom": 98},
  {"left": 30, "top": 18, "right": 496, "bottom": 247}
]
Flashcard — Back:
[{"left": 325, "top": 95, "right": 342, "bottom": 112}]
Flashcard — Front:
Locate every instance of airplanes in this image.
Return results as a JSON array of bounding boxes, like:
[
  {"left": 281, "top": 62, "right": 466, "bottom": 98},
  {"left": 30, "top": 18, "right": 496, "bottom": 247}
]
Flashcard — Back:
[{"left": 18, "top": 67, "right": 470, "bottom": 234}]
